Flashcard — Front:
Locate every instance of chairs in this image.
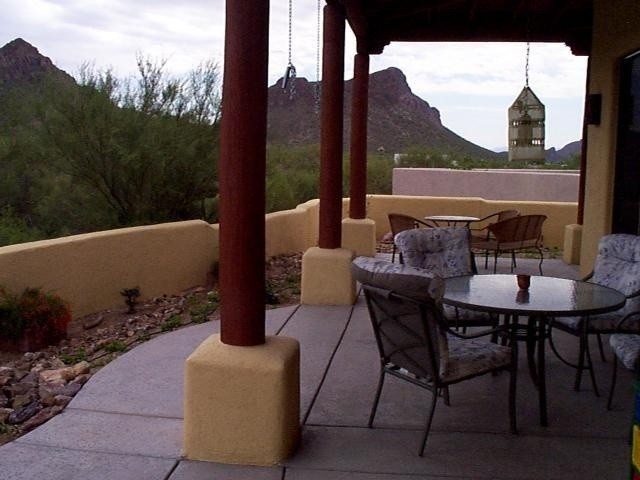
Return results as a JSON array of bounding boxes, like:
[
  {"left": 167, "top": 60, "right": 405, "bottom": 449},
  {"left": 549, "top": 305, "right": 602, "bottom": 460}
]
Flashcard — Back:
[
  {"left": 352, "top": 256, "right": 518, "bottom": 456},
  {"left": 388, "top": 208, "right": 434, "bottom": 264},
  {"left": 487, "top": 214, "right": 548, "bottom": 276},
  {"left": 466, "top": 209, "right": 521, "bottom": 270},
  {"left": 549, "top": 234, "right": 640, "bottom": 392},
  {"left": 394, "top": 226, "right": 500, "bottom": 343},
  {"left": 606, "top": 311, "right": 640, "bottom": 411}
]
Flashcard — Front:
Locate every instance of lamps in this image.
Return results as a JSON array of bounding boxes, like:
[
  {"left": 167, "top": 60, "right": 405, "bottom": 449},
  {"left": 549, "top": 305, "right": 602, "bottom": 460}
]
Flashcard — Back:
[{"left": 509, "top": 42, "right": 545, "bottom": 161}]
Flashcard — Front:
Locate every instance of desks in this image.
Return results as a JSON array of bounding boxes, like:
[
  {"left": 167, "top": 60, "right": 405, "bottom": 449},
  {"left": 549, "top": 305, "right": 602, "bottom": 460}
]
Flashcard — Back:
[
  {"left": 439, "top": 273, "right": 626, "bottom": 428},
  {"left": 424, "top": 216, "right": 480, "bottom": 227}
]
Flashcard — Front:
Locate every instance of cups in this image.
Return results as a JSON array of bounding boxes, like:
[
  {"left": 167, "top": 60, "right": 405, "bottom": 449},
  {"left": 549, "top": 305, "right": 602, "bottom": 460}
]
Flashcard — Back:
[
  {"left": 516, "top": 289, "right": 530, "bottom": 304},
  {"left": 517, "top": 274, "right": 530, "bottom": 288}
]
